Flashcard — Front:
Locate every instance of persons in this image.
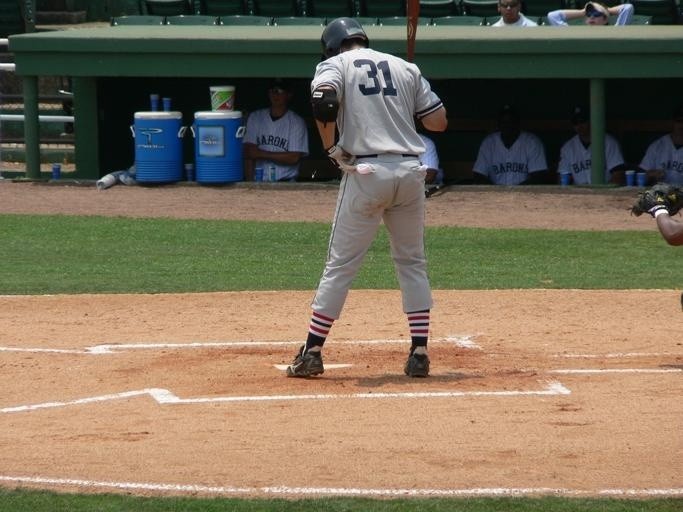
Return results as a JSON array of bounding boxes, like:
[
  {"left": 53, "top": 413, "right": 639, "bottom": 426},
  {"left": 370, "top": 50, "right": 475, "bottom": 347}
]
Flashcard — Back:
[
  {"left": 415, "top": 132, "right": 444, "bottom": 184},
  {"left": 636, "top": 113, "right": 682, "bottom": 189},
  {"left": 633, "top": 182, "right": 683, "bottom": 306},
  {"left": 547, "top": 0, "right": 635, "bottom": 25},
  {"left": 556, "top": 111, "right": 625, "bottom": 185},
  {"left": 287, "top": 18, "right": 447, "bottom": 376},
  {"left": 243, "top": 78, "right": 310, "bottom": 182},
  {"left": 490, "top": 1, "right": 539, "bottom": 26},
  {"left": 472, "top": 104, "right": 548, "bottom": 185}
]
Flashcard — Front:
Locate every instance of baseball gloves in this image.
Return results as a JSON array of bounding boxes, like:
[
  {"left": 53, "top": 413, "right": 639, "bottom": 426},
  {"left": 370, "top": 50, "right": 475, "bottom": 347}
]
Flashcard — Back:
[{"left": 634, "top": 181, "right": 681, "bottom": 217}]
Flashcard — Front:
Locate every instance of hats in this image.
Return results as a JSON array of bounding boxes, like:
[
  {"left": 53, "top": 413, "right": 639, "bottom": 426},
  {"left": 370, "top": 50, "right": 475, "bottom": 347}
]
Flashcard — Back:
[
  {"left": 582, "top": 2, "right": 610, "bottom": 20},
  {"left": 267, "top": 78, "right": 289, "bottom": 93}
]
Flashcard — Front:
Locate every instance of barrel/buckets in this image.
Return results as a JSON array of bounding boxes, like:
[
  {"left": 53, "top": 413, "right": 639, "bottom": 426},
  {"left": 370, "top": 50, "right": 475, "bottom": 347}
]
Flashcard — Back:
[
  {"left": 190, "top": 110, "right": 246, "bottom": 184},
  {"left": 209, "top": 85, "right": 236, "bottom": 113},
  {"left": 130, "top": 111, "right": 188, "bottom": 185}
]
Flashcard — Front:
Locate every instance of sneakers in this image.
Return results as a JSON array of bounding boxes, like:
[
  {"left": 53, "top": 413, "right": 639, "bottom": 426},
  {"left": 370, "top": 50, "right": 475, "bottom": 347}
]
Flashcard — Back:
[
  {"left": 285, "top": 344, "right": 323, "bottom": 377},
  {"left": 404, "top": 347, "right": 429, "bottom": 376}
]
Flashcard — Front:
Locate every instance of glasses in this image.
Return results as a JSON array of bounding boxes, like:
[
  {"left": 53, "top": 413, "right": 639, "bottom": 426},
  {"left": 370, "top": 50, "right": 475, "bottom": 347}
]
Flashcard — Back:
[
  {"left": 584, "top": 10, "right": 603, "bottom": 18},
  {"left": 498, "top": 1, "right": 519, "bottom": 9}
]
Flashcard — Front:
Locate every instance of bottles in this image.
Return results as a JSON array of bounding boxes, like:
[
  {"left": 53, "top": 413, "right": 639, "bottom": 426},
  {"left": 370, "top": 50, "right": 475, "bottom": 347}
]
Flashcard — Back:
[
  {"left": 268, "top": 165, "right": 275, "bottom": 182},
  {"left": 51, "top": 163, "right": 60, "bottom": 178}
]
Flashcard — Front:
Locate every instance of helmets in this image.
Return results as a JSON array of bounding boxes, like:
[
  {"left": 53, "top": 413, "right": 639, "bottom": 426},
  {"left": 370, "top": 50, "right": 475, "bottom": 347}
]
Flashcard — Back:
[{"left": 320, "top": 18, "right": 369, "bottom": 57}]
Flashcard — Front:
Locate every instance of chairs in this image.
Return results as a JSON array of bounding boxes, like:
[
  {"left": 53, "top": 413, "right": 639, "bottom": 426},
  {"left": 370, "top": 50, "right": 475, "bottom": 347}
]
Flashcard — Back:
[{"left": 88, "top": 0, "right": 678, "bottom": 27}]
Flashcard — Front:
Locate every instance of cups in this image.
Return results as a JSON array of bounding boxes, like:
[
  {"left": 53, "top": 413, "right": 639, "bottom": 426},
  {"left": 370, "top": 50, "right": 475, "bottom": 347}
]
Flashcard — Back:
[
  {"left": 150, "top": 94, "right": 158, "bottom": 111},
  {"left": 162, "top": 98, "right": 172, "bottom": 112},
  {"left": 559, "top": 172, "right": 571, "bottom": 185},
  {"left": 183, "top": 164, "right": 193, "bottom": 179},
  {"left": 635, "top": 173, "right": 648, "bottom": 187},
  {"left": 625, "top": 171, "right": 635, "bottom": 187},
  {"left": 254, "top": 168, "right": 264, "bottom": 180}
]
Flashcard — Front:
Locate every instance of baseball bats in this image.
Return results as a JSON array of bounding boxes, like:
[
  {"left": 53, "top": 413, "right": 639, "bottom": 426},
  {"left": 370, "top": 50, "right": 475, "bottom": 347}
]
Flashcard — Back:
[
  {"left": 425, "top": 178, "right": 456, "bottom": 197},
  {"left": 406, "top": 0, "right": 420, "bottom": 62}
]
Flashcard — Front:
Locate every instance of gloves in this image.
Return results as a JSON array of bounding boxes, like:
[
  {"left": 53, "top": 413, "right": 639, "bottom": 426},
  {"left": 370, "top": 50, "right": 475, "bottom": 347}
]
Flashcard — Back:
[{"left": 325, "top": 143, "right": 358, "bottom": 172}]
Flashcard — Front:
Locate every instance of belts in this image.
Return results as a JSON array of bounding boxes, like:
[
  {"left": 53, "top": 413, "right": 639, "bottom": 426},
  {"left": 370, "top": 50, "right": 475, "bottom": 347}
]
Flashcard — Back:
[{"left": 358, "top": 153, "right": 421, "bottom": 158}]
